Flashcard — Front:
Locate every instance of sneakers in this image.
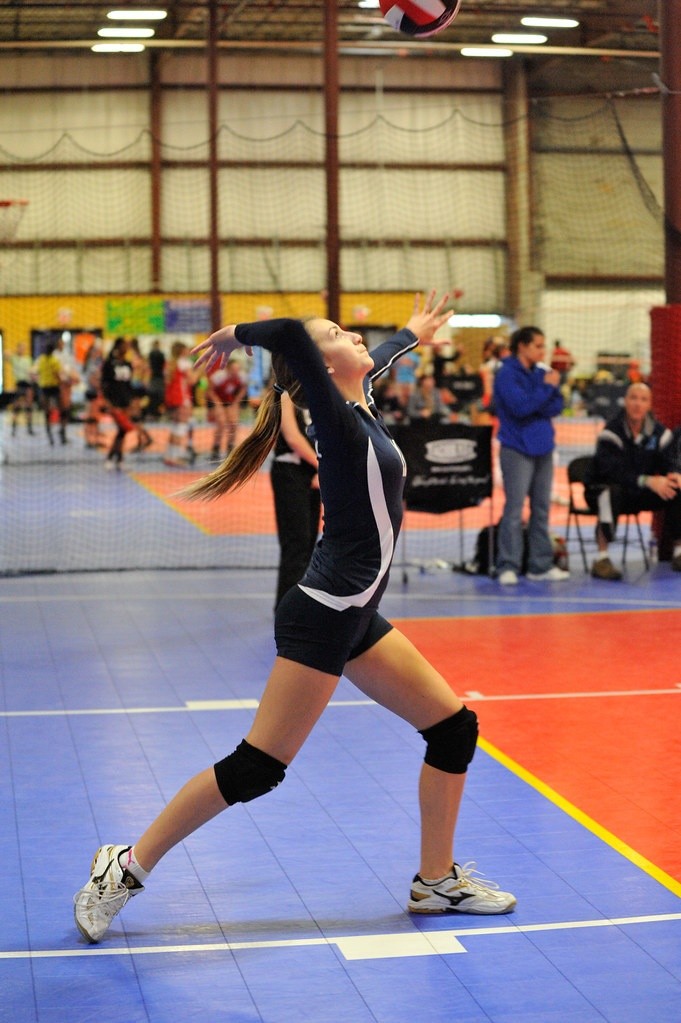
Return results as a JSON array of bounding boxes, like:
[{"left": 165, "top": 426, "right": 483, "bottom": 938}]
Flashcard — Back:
[
  {"left": 75, "top": 844, "right": 142, "bottom": 942},
  {"left": 407, "top": 865, "right": 515, "bottom": 916}
]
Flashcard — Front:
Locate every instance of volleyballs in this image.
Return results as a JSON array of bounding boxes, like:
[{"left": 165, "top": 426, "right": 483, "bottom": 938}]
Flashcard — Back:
[{"left": 379, "top": 0, "right": 462, "bottom": 38}]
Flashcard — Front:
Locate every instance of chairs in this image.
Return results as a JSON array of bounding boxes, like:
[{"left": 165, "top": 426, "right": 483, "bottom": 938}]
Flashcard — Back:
[
  {"left": 564, "top": 454, "right": 651, "bottom": 572},
  {"left": 386, "top": 421, "right": 495, "bottom": 584}
]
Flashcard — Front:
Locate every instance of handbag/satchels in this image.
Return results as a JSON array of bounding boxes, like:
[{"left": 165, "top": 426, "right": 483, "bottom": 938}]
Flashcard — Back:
[{"left": 477, "top": 522, "right": 531, "bottom": 576}]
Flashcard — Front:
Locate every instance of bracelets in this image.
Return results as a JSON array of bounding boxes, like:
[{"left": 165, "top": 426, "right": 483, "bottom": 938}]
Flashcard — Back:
[{"left": 638, "top": 475, "right": 647, "bottom": 487}]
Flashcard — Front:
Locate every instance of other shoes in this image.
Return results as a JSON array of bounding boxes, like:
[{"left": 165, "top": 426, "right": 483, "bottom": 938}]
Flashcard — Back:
[
  {"left": 590, "top": 558, "right": 621, "bottom": 580},
  {"left": 499, "top": 572, "right": 519, "bottom": 586},
  {"left": 672, "top": 554, "right": 681, "bottom": 572},
  {"left": 528, "top": 567, "right": 569, "bottom": 582}
]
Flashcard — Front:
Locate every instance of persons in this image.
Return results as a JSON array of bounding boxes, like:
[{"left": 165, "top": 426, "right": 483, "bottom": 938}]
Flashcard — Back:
[
  {"left": 431, "top": 346, "right": 462, "bottom": 375},
  {"left": 493, "top": 326, "right": 571, "bottom": 587},
  {"left": 163, "top": 343, "right": 206, "bottom": 466},
  {"left": 129, "top": 339, "right": 166, "bottom": 419},
  {"left": 550, "top": 341, "right": 576, "bottom": 382},
  {"left": 268, "top": 407, "right": 321, "bottom": 602},
  {"left": 102, "top": 337, "right": 135, "bottom": 470},
  {"left": 74, "top": 289, "right": 517, "bottom": 943},
  {"left": 584, "top": 383, "right": 681, "bottom": 580},
  {"left": 405, "top": 374, "right": 450, "bottom": 424},
  {"left": 4, "top": 332, "right": 106, "bottom": 448},
  {"left": 479, "top": 335, "right": 511, "bottom": 437},
  {"left": 206, "top": 361, "right": 247, "bottom": 465},
  {"left": 377, "top": 367, "right": 402, "bottom": 412}
]
